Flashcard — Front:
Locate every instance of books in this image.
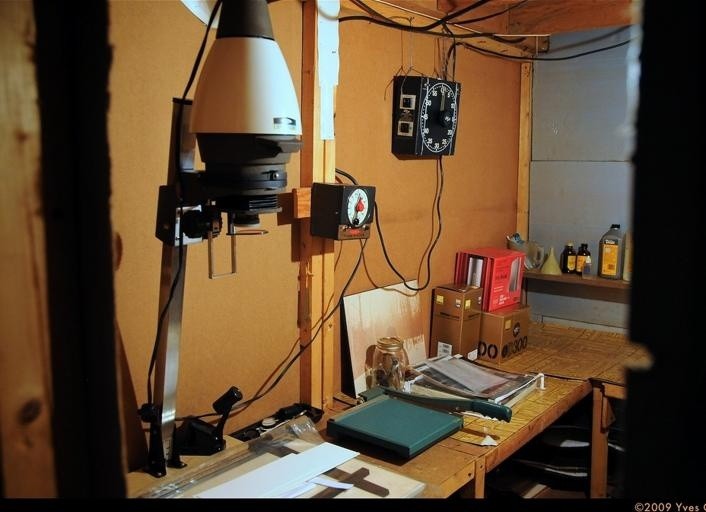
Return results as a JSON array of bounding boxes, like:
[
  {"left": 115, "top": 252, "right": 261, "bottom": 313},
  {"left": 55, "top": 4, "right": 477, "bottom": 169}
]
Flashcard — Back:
[{"left": 411, "top": 355, "right": 537, "bottom": 409}]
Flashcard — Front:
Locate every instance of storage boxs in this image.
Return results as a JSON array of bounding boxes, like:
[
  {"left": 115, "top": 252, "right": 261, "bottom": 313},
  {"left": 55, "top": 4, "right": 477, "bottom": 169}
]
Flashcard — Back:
[{"left": 429, "top": 247, "right": 531, "bottom": 365}]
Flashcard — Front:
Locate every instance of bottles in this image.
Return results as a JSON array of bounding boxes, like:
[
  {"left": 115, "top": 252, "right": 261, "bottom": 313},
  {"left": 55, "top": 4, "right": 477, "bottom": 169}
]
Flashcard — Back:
[
  {"left": 597, "top": 224, "right": 625, "bottom": 280},
  {"left": 559, "top": 240, "right": 576, "bottom": 274},
  {"left": 373, "top": 336, "right": 410, "bottom": 394},
  {"left": 536, "top": 246, "right": 562, "bottom": 276},
  {"left": 582, "top": 255, "right": 594, "bottom": 280},
  {"left": 575, "top": 243, "right": 591, "bottom": 277}
]
